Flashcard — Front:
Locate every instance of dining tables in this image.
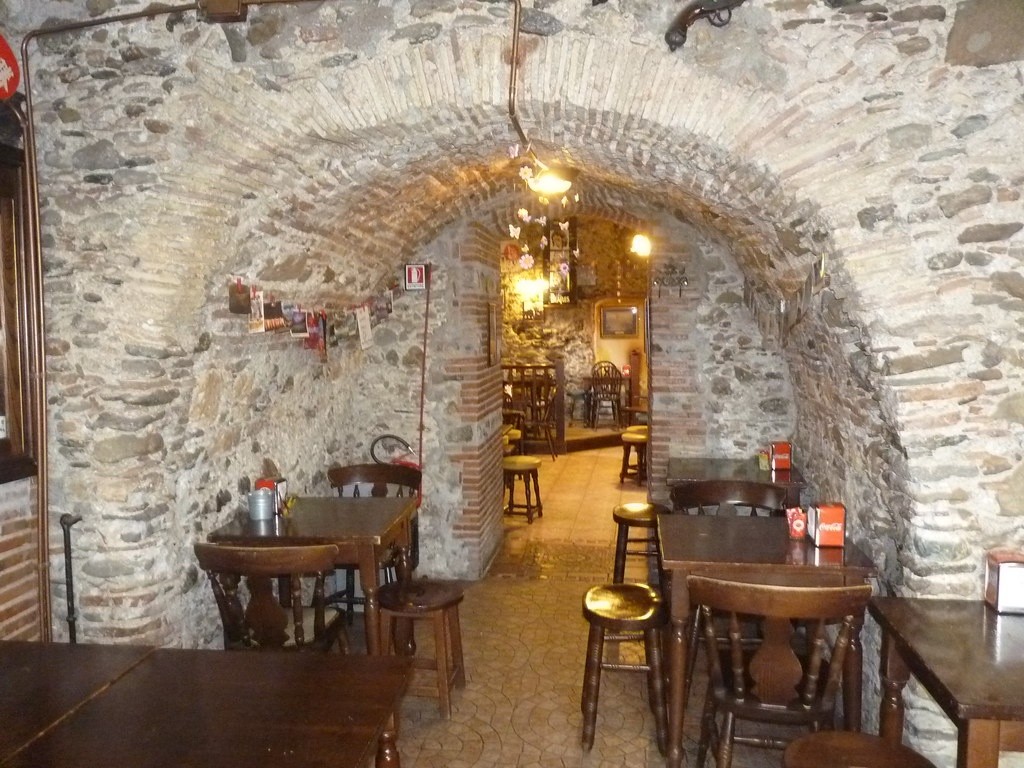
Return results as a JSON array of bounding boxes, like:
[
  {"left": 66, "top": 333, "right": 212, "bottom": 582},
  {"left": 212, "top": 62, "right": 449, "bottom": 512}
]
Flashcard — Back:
[
  {"left": 0, "top": 641, "right": 160, "bottom": 767},
  {"left": 668, "top": 457, "right": 809, "bottom": 514},
  {"left": 582, "top": 376, "right": 633, "bottom": 427},
  {"left": 866, "top": 597, "right": 1024, "bottom": 768},
  {"left": 206, "top": 497, "right": 418, "bottom": 655},
  {"left": 654, "top": 512, "right": 879, "bottom": 768},
  {"left": 0, "top": 648, "right": 416, "bottom": 768}
]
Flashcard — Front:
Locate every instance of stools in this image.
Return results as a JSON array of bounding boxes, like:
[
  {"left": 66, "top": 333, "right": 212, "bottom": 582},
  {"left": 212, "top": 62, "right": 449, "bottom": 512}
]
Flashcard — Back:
[
  {"left": 375, "top": 578, "right": 467, "bottom": 721},
  {"left": 627, "top": 426, "right": 648, "bottom": 433},
  {"left": 581, "top": 582, "right": 670, "bottom": 756},
  {"left": 567, "top": 390, "right": 586, "bottom": 429},
  {"left": 612, "top": 502, "right": 671, "bottom": 590},
  {"left": 781, "top": 729, "right": 934, "bottom": 768},
  {"left": 502, "top": 455, "right": 543, "bottom": 525},
  {"left": 620, "top": 433, "right": 648, "bottom": 488}
]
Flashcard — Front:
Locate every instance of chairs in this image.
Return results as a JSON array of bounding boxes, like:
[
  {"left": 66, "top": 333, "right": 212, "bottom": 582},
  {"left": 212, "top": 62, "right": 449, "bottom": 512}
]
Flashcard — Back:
[
  {"left": 685, "top": 575, "right": 873, "bottom": 767},
  {"left": 501, "top": 364, "right": 557, "bottom": 461},
  {"left": 590, "top": 360, "right": 617, "bottom": 376},
  {"left": 669, "top": 480, "right": 788, "bottom": 516},
  {"left": 311, "top": 464, "right": 423, "bottom": 625},
  {"left": 591, "top": 365, "right": 623, "bottom": 429},
  {"left": 193, "top": 542, "right": 351, "bottom": 650}
]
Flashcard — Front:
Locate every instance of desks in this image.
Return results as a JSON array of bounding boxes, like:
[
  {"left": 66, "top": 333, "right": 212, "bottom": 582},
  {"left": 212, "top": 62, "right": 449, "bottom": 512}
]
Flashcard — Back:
[{"left": 501, "top": 375, "right": 556, "bottom": 439}]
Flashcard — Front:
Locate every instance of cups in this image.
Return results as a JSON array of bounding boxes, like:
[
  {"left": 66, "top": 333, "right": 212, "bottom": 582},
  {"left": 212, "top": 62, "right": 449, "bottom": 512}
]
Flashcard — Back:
[{"left": 247, "top": 489, "right": 273, "bottom": 520}]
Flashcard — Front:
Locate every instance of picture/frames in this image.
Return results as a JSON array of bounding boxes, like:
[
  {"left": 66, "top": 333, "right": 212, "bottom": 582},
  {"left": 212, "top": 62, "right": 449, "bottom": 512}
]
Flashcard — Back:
[{"left": 600, "top": 304, "right": 638, "bottom": 339}]
